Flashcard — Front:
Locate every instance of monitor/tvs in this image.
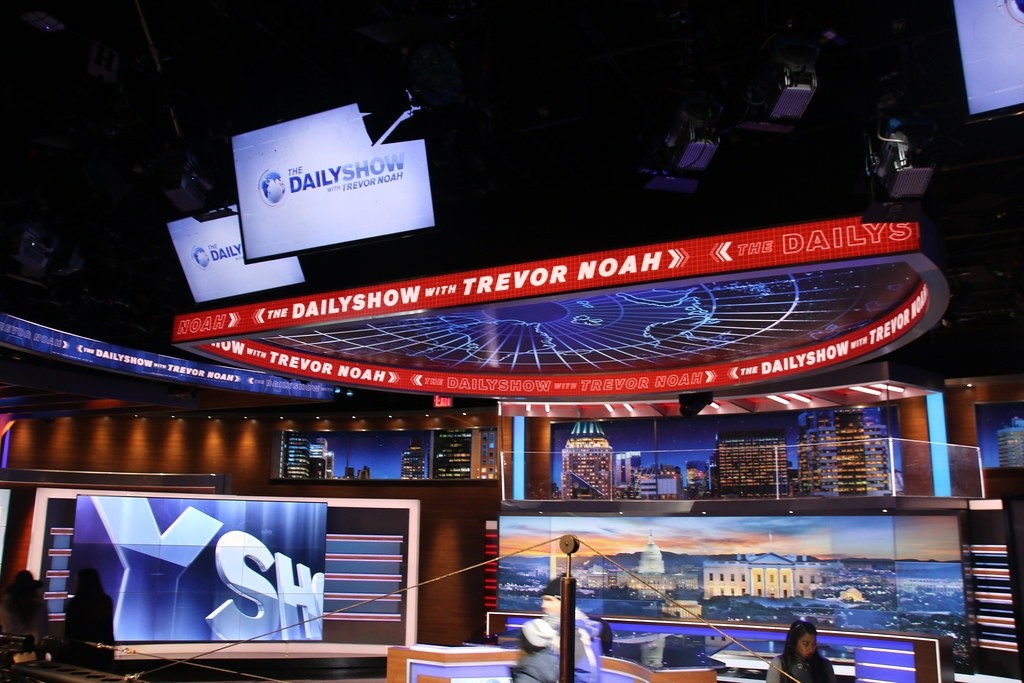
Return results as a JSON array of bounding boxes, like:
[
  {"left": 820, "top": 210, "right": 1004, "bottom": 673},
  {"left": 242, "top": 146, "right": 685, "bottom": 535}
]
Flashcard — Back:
[
  {"left": 951, "top": 0, "right": 1024, "bottom": 123},
  {"left": 166, "top": 203, "right": 311, "bottom": 306},
  {"left": 229, "top": 97, "right": 442, "bottom": 265}
]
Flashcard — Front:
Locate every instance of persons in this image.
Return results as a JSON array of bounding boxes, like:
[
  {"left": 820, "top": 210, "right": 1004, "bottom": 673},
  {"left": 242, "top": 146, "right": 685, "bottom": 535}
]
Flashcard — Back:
[
  {"left": 0, "top": 569, "right": 50, "bottom": 645},
  {"left": 64, "top": 568, "right": 114, "bottom": 673},
  {"left": 512, "top": 619, "right": 559, "bottom": 683},
  {"left": 767, "top": 620, "right": 836, "bottom": 683},
  {"left": 541, "top": 573, "right": 603, "bottom": 683}
]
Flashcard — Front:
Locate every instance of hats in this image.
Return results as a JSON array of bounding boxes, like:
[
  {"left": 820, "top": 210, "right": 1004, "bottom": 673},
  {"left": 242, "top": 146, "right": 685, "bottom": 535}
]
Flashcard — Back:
[{"left": 7, "top": 570, "right": 45, "bottom": 596}]
